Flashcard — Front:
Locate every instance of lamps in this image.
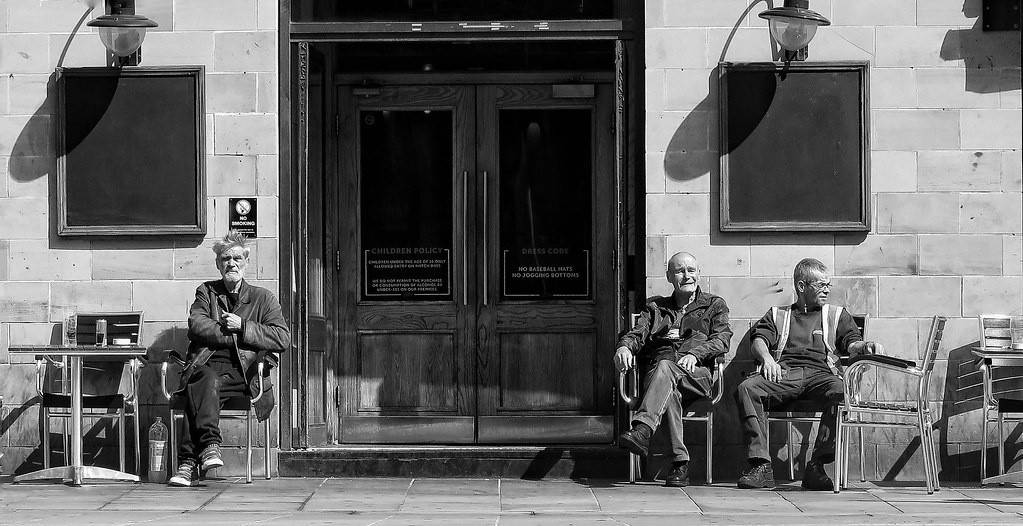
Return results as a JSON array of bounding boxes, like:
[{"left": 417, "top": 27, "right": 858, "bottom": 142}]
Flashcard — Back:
[
  {"left": 86, "top": 0, "right": 158, "bottom": 69},
  {"left": 757, "top": 0, "right": 832, "bottom": 74}
]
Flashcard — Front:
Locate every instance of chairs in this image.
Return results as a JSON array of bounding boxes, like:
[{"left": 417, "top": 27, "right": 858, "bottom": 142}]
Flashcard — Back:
[
  {"left": 745, "top": 313, "right": 868, "bottom": 490},
  {"left": 833, "top": 313, "right": 947, "bottom": 495},
  {"left": 159, "top": 348, "right": 280, "bottom": 486},
  {"left": 977, "top": 313, "right": 1023, "bottom": 486},
  {"left": 619, "top": 312, "right": 726, "bottom": 487},
  {"left": 35, "top": 310, "right": 146, "bottom": 473}
]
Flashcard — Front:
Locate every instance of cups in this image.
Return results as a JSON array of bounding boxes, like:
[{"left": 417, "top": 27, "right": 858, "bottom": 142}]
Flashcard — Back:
[
  {"left": 1010, "top": 317, "right": 1023, "bottom": 350},
  {"left": 113, "top": 338, "right": 131, "bottom": 349},
  {"left": 96, "top": 319, "right": 106, "bottom": 347}
]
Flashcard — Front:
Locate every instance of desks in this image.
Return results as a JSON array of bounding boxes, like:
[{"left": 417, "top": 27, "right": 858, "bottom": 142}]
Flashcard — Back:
[
  {"left": 6, "top": 343, "right": 147, "bottom": 487},
  {"left": 970, "top": 344, "right": 1023, "bottom": 489}
]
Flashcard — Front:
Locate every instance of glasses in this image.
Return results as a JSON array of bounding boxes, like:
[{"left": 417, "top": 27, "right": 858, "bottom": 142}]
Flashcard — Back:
[{"left": 802, "top": 280, "right": 833, "bottom": 290}]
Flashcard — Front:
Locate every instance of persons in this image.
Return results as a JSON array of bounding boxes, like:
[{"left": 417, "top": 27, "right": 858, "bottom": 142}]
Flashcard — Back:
[
  {"left": 612, "top": 252, "right": 733, "bottom": 487},
  {"left": 737, "top": 258, "right": 886, "bottom": 491},
  {"left": 168, "top": 232, "right": 291, "bottom": 487}
]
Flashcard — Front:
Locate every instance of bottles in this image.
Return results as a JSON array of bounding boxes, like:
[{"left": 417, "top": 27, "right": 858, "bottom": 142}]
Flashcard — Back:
[
  {"left": 62, "top": 306, "right": 77, "bottom": 347},
  {"left": 147, "top": 417, "right": 168, "bottom": 483}
]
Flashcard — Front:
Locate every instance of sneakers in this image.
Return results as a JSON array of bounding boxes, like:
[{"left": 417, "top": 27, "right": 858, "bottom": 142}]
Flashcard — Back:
[
  {"left": 169, "top": 463, "right": 199, "bottom": 486},
  {"left": 802, "top": 461, "right": 833, "bottom": 490},
  {"left": 738, "top": 462, "right": 775, "bottom": 488},
  {"left": 198, "top": 444, "right": 223, "bottom": 471}
]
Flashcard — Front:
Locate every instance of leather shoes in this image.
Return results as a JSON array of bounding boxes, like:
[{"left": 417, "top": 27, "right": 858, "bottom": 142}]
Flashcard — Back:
[
  {"left": 619, "top": 424, "right": 650, "bottom": 457},
  {"left": 665, "top": 461, "right": 688, "bottom": 486}
]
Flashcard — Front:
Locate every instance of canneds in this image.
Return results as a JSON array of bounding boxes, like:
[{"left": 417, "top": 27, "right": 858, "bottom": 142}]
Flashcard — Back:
[{"left": 96, "top": 319, "right": 107, "bottom": 346}]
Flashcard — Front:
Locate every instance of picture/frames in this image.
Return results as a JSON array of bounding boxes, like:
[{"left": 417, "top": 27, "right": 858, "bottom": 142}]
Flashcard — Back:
[
  {"left": 54, "top": 64, "right": 208, "bottom": 237},
  {"left": 717, "top": 61, "right": 871, "bottom": 234}
]
